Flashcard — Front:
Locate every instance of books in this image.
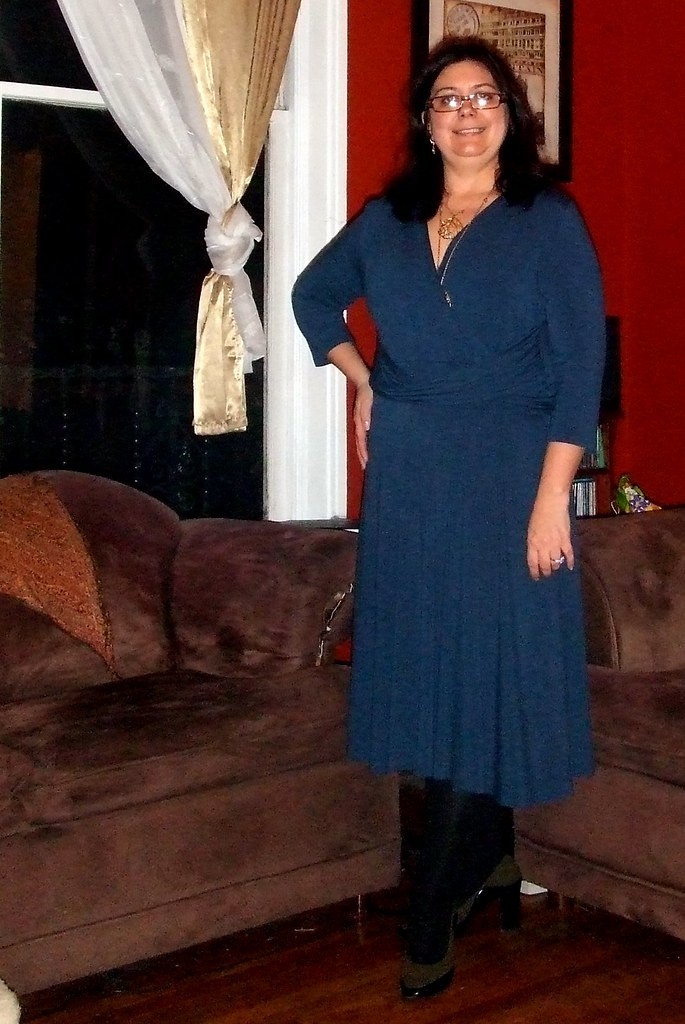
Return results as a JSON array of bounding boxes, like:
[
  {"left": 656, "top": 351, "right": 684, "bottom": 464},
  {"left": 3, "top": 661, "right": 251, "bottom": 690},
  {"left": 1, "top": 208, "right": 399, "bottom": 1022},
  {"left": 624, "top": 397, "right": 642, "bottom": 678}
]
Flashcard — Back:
[{"left": 570, "top": 424, "right": 606, "bottom": 517}]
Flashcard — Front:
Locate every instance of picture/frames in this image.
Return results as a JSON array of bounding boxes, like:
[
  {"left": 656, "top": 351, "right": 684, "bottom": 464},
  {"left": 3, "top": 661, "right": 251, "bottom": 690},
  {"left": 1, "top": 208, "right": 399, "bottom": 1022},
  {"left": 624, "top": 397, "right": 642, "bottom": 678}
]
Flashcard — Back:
[{"left": 410, "top": 0, "right": 574, "bottom": 182}]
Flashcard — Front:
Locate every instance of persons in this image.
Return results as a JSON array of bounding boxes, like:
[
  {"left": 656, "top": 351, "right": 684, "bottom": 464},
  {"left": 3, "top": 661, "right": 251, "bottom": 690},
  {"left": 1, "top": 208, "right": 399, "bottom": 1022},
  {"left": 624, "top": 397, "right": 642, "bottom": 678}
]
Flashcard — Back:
[{"left": 292, "top": 33, "right": 606, "bottom": 1000}]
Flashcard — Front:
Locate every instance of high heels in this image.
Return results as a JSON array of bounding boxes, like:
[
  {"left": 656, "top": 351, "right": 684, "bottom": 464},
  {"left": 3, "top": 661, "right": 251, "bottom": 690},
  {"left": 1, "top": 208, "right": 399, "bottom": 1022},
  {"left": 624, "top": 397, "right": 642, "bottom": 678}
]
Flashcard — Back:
[
  {"left": 396, "top": 854, "right": 523, "bottom": 935},
  {"left": 400, "top": 913, "right": 458, "bottom": 996}
]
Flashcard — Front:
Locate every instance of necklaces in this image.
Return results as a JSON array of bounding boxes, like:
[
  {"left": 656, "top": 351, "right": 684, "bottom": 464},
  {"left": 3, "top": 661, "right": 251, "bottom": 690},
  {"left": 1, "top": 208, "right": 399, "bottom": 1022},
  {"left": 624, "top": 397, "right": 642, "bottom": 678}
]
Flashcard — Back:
[{"left": 436, "top": 188, "right": 494, "bottom": 307}]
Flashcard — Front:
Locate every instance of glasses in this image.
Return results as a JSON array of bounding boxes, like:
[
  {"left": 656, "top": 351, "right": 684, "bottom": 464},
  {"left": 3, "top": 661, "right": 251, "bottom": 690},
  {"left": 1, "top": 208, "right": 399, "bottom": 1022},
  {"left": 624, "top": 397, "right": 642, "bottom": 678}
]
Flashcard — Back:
[{"left": 425, "top": 92, "right": 506, "bottom": 113}]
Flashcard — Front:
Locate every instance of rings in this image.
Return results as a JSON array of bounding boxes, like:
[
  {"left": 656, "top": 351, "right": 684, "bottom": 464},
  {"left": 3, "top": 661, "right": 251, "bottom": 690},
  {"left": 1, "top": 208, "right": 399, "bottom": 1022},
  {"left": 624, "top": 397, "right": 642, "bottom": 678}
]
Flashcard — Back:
[{"left": 550, "top": 556, "right": 565, "bottom": 566}]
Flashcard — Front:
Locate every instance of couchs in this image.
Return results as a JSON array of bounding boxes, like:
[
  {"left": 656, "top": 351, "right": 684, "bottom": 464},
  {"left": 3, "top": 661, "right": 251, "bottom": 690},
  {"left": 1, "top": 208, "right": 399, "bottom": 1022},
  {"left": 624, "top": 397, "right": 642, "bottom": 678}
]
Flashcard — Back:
[{"left": 0, "top": 469, "right": 685, "bottom": 999}]
